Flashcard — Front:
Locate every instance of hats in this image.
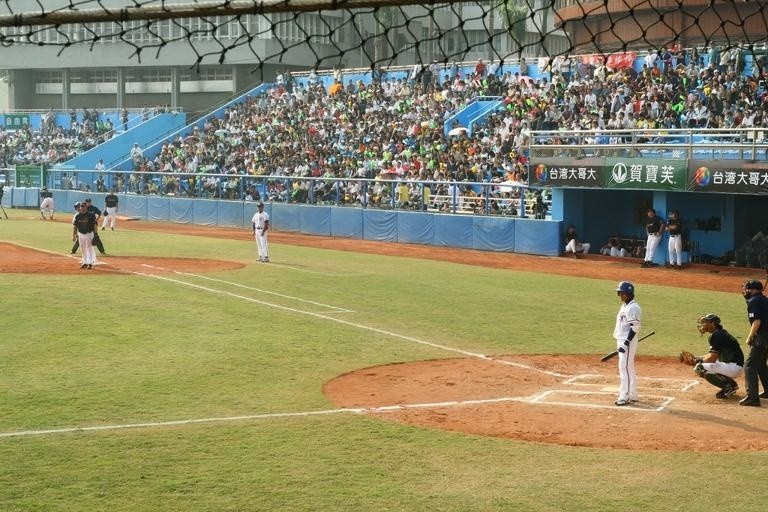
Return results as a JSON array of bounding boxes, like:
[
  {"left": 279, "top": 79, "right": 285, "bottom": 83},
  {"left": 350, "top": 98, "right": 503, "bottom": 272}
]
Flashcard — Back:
[
  {"left": 74, "top": 199, "right": 91, "bottom": 210},
  {"left": 257, "top": 203, "right": 264, "bottom": 207}
]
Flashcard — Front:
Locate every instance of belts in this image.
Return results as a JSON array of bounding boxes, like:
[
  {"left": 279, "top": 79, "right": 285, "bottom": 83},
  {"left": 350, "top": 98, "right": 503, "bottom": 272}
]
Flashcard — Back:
[
  {"left": 671, "top": 235, "right": 678, "bottom": 237},
  {"left": 257, "top": 228, "right": 262, "bottom": 229},
  {"left": 650, "top": 233, "right": 657, "bottom": 235}
]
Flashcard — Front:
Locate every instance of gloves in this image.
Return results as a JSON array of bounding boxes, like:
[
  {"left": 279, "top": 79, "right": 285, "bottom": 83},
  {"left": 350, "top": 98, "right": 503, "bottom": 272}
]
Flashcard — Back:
[{"left": 619, "top": 347, "right": 625, "bottom": 353}]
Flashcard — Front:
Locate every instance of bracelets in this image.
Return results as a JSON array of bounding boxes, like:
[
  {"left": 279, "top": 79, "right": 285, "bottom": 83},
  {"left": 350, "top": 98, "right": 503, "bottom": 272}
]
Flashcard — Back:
[
  {"left": 263, "top": 230, "right": 265, "bottom": 232},
  {"left": 695, "top": 358, "right": 704, "bottom": 363}
]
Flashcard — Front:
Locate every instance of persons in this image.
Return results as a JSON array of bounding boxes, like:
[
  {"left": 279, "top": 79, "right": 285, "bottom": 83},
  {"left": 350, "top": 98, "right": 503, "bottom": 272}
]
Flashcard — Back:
[
  {"left": 68, "top": 197, "right": 110, "bottom": 257},
  {"left": 100, "top": 190, "right": 118, "bottom": 231},
  {"left": 639, "top": 208, "right": 665, "bottom": 267},
  {"left": 0, "top": 36, "right": 767, "bottom": 221},
  {"left": 737, "top": 278, "right": 768, "bottom": 406},
  {"left": 562, "top": 223, "right": 591, "bottom": 259},
  {"left": 612, "top": 280, "right": 642, "bottom": 406},
  {"left": 72, "top": 200, "right": 98, "bottom": 270},
  {"left": 679, "top": 312, "right": 744, "bottom": 398},
  {"left": 250, "top": 201, "right": 270, "bottom": 263},
  {"left": 599, "top": 232, "right": 648, "bottom": 259},
  {"left": 662, "top": 207, "right": 683, "bottom": 269},
  {"left": 736, "top": 224, "right": 768, "bottom": 268}
]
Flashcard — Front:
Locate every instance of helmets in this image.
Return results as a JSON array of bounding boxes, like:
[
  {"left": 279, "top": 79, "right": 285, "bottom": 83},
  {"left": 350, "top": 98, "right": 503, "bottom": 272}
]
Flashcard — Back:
[
  {"left": 697, "top": 314, "right": 720, "bottom": 334},
  {"left": 41, "top": 186, "right": 48, "bottom": 191},
  {"left": 617, "top": 282, "right": 634, "bottom": 296},
  {"left": 742, "top": 280, "right": 763, "bottom": 300}
]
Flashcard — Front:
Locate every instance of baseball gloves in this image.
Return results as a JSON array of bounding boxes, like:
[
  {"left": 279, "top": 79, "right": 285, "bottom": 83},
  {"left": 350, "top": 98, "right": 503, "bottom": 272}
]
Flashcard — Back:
[{"left": 680, "top": 349, "right": 697, "bottom": 366}]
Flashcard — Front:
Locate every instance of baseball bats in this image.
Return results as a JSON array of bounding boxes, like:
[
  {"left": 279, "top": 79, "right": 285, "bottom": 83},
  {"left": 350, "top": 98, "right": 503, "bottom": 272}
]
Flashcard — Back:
[{"left": 601, "top": 332, "right": 655, "bottom": 361}]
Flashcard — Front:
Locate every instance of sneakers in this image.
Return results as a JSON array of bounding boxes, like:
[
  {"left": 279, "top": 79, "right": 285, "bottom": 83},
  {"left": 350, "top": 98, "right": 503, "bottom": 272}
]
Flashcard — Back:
[
  {"left": 102, "top": 251, "right": 105, "bottom": 254},
  {"left": 102, "top": 227, "right": 114, "bottom": 231},
  {"left": 639, "top": 261, "right": 682, "bottom": 270},
  {"left": 70, "top": 251, "right": 75, "bottom": 254},
  {"left": 614, "top": 397, "right": 630, "bottom": 405},
  {"left": 80, "top": 261, "right": 94, "bottom": 269},
  {"left": 716, "top": 383, "right": 768, "bottom": 405},
  {"left": 256, "top": 256, "right": 269, "bottom": 262}
]
